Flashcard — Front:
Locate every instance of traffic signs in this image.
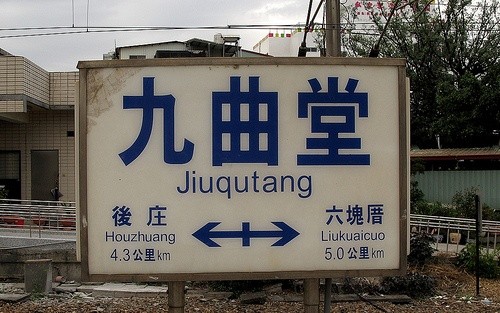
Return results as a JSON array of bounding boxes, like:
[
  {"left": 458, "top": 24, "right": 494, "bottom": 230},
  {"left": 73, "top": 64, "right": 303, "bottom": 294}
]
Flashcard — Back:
[{"left": 75, "top": 58, "right": 409, "bottom": 276}]
